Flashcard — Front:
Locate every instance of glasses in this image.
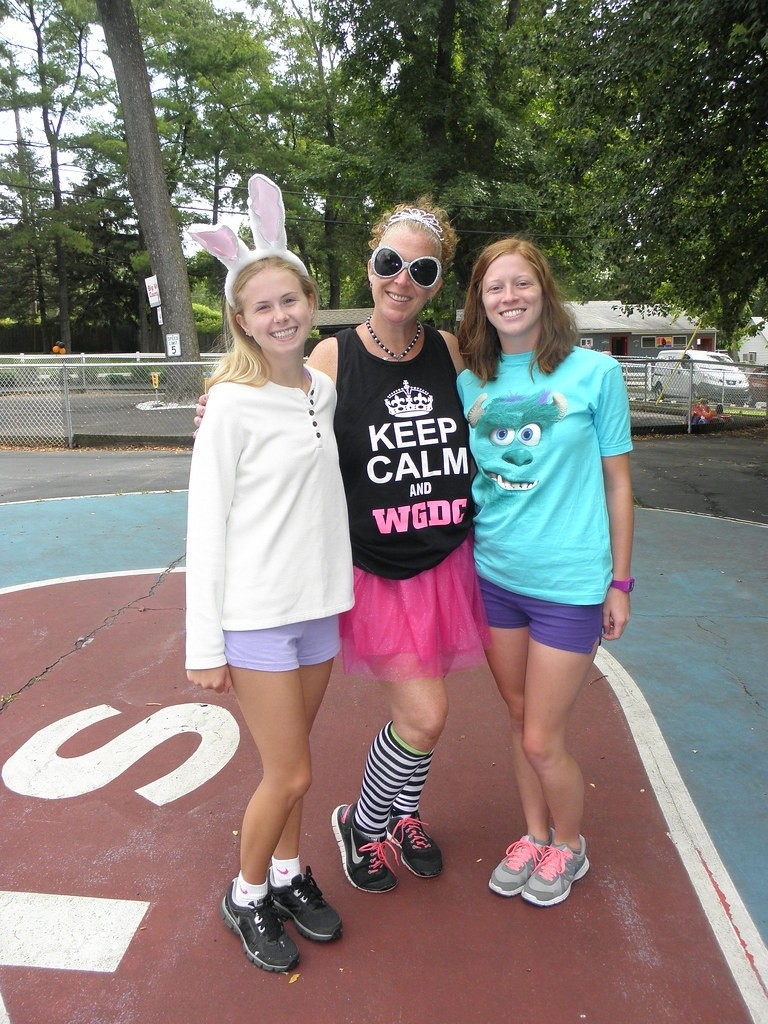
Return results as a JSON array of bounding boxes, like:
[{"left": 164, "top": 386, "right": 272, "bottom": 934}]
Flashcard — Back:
[{"left": 371, "top": 245, "right": 444, "bottom": 289}]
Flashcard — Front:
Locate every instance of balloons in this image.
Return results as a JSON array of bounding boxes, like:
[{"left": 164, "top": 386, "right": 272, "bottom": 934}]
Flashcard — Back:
[{"left": 52, "top": 341, "right": 66, "bottom": 354}]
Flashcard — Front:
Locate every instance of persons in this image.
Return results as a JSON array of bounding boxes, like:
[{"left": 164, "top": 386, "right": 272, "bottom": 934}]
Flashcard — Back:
[
  {"left": 185, "top": 247, "right": 357, "bottom": 973},
  {"left": 683, "top": 398, "right": 723, "bottom": 426},
  {"left": 455, "top": 238, "right": 637, "bottom": 907},
  {"left": 193, "top": 197, "right": 468, "bottom": 893}
]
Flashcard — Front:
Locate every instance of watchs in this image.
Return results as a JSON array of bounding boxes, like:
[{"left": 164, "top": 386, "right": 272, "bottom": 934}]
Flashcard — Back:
[{"left": 610, "top": 578, "right": 635, "bottom": 592}]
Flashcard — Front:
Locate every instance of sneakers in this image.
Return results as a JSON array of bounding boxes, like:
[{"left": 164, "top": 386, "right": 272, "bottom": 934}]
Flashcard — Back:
[
  {"left": 330, "top": 802, "right": 398, "bottom": 893},
  {"left": 219, "top": 877, "right": 300, "bottom": 972},
  {"left": 521, "top": 832, "right": 591, "bottom": 908},
  {"left": 266, "top": 864, "right": 343, "bottom": 941},
  {"left": 386, "top": 810, "right": 443, "bottom": 878},
  {"left": 488, "top": 824, "right": 556, "bottom": 897}
]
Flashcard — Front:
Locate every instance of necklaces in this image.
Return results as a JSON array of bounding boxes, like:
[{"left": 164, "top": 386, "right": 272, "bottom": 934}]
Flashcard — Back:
[{"left": 367, "top": 314, "right": 421, "bottom": 361}]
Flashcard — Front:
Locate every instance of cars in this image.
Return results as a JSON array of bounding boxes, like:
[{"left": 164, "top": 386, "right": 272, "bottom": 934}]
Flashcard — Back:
[{"left": 747, "top": 367, "right": 768, "bottom": 405}]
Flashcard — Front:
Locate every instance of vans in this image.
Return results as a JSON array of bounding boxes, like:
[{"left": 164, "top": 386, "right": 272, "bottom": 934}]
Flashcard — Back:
[{"left": 651, "top": 349, "right": 750, "bottom": 408}]
